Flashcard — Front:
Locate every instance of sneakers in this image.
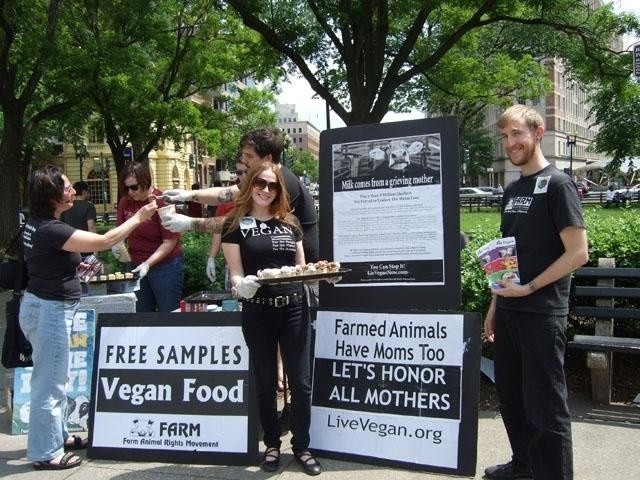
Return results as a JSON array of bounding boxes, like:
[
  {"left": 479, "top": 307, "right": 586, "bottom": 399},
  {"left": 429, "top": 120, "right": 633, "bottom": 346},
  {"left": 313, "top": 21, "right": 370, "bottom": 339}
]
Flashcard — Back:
[{"left": 484, "top": 456, "right": 515, "bottom": 477}]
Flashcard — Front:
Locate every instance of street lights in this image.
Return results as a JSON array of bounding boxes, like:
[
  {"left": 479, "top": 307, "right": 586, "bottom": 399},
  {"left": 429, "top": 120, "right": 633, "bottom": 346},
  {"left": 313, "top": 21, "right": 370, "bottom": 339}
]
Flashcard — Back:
[
  {"left": 281, "top": 132, "right": 294, "bottom": 166},
  {"left": 565, "top": 130, "right": 579, "bottom": 177},
  {"left": 93, "top": 152, "right": 111, "bottom": 223}
]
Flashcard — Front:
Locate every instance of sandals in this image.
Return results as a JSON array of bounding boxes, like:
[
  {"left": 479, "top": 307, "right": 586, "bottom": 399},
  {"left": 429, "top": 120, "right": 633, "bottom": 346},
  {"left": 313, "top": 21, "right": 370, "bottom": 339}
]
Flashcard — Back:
[
  {"left": 35, "top": 451, "right": 81, "bottom": 469},
  {"left": 63, "top": 434, "right": 88, "bottom": 450},
  {"left": 259, "top": 445, "right": 280, "bottom": 472},
  {"left": 294, "top": 450, "right": 321, "bottom": 475}
]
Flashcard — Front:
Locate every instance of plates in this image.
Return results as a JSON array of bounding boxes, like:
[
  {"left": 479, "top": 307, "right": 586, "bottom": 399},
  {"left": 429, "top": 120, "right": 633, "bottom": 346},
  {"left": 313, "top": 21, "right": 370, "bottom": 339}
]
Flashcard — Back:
[
  {"left": 254, "top": 268, "right": 353, "bottom": 283},
  {"left": 80, "top": 274, "right": 139, "bottom": 285}
]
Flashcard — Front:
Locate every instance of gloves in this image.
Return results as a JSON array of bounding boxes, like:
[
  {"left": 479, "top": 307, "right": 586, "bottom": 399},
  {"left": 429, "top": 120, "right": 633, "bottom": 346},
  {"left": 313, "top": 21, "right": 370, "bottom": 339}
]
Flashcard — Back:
[
  {"left": 160, "top": 212, "right": 192, "bottom": 233},
  {"left": 132, "top": 263, "right": 150, "bottom": 280},
  {"left": 111, "top": 246, "right": 120, "bottom": 259},
  {"left": 163, "top": 187, "right": 192, "bottom": 204},
  {"left": 206, "top": 257, "right": 216, "bottom": 282}
]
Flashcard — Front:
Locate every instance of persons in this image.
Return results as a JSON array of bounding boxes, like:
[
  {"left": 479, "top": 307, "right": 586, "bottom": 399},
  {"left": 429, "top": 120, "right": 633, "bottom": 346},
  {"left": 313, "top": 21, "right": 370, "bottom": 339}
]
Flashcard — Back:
[
  {"left": 205, "top": 150, "right": 289, "bottom": 391},
  {"left": 620, "top": 186, "right": 633, "bottom": 206},
  {"left": 207, "top": 179, "right": 222, "bottom": 217},
  {"left": 182, "top": 183, "right": 205, "bottom": 217},
  {"left": 219, "top": 161, "right": 343, "bottom": 476},
  {"left": 605, "top": 185, "right": 620, "bottom": 209},
  {"left": 18, "top": 166, "right": 158, "bottom": 471},
  {"left": 59, "top": 181, "right": 99, "bottom": 262},
  {"left": 483, "top": 104, "right": 590, "bottom": 480},
  {"left": 160, "top": 128, "right": 320, "bottom": 438},
  {"left": 111, "top": 161, "right": 186, "bottom": 312}
]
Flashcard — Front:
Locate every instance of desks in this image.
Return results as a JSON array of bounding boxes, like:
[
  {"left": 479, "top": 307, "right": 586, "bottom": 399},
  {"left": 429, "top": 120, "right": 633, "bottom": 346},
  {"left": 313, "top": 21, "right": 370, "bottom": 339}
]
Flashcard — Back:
[{"left": 10, "top": 291, "right": 138, "bottom": 438}]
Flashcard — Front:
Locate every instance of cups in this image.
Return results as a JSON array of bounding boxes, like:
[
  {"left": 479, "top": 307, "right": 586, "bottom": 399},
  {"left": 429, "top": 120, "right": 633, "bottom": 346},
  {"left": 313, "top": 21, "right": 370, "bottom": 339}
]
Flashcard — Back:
[{"left": 157, "top": 205, "right": 176, "bottom": 221}]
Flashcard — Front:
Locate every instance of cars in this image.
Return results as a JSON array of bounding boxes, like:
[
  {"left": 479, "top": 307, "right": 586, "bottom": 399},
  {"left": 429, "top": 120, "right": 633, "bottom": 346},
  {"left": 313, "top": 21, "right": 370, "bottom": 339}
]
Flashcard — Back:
[{"left": 460, "top": 188, "right": 492, "bottom": 201}]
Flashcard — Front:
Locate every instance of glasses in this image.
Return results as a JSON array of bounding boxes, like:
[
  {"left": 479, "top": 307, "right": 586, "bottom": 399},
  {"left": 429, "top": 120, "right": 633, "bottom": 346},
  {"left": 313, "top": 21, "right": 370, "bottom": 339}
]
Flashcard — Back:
[
  {"left": 252, "top": 177, "right": 280, "bottom": 194},
  {"left": 235, "top": 168, "right": 248, "bottom": 176},
  {"left": 124, "top": 183, "right": 140, "bottom": 192}
]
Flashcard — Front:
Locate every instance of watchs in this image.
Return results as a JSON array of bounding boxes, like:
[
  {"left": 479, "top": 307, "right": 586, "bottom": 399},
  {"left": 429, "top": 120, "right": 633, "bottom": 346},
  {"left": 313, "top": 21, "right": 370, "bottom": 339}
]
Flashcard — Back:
[{"left": 528, "top": 281, "right": 537, "bottom": 294}]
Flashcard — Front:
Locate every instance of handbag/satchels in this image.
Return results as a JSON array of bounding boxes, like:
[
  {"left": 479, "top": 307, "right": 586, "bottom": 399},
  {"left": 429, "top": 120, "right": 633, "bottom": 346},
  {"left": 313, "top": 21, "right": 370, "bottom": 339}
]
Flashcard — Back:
[{"left": 1, "top": 250, "right": 34, "bottom": 369}]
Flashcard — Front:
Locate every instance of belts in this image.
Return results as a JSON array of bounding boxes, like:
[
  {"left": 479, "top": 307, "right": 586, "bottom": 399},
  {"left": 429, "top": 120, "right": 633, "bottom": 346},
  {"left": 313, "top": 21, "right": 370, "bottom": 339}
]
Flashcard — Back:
[{"left": 239, "top": 292, "right": 303, "bottom": 308}]
